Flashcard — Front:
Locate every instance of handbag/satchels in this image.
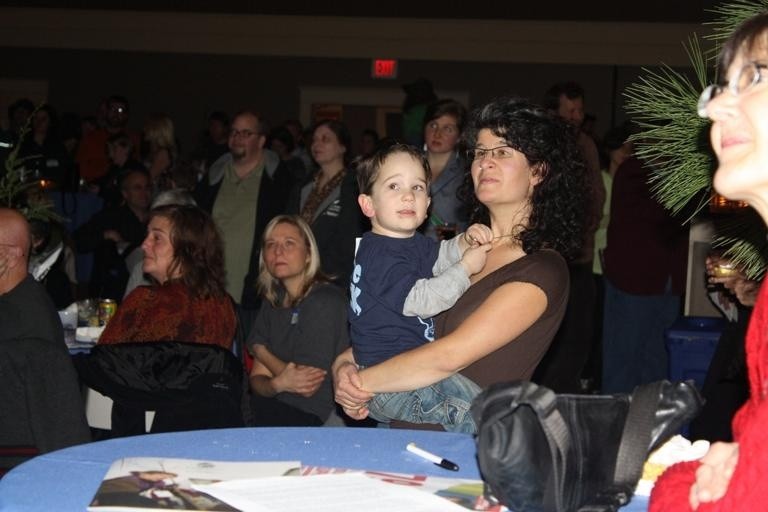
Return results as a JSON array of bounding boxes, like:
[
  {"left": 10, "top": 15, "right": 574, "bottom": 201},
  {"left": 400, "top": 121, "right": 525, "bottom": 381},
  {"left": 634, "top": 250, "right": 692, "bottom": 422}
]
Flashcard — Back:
[{"left": 478, "top": 379, "right": 698, "bottom": 512}]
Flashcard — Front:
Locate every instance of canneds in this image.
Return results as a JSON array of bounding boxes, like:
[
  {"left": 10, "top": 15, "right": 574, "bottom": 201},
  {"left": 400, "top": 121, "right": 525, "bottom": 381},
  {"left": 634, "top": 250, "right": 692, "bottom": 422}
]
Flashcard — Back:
[{"left": 99, "top": 299, "right": 117, "bottom": 327}]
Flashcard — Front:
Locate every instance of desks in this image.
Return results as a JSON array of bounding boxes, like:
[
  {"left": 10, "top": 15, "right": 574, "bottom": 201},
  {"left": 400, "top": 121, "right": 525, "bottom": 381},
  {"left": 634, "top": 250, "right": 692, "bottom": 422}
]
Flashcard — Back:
[{"left": 0, "top": 426, "right": 656, "bottom": 512}]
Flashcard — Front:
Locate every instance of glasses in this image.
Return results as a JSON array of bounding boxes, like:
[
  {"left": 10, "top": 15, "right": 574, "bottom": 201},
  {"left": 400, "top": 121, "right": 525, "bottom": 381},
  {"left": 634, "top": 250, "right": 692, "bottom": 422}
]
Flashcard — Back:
[
  {"left": 228, "top": 129, "right": 259, "bottom": 137},
  {"left": 697, "top": 62, "right": 768, "bottom": 119},
  {"left": 465, "top": 146, "right": 514, "bottom": 160}
]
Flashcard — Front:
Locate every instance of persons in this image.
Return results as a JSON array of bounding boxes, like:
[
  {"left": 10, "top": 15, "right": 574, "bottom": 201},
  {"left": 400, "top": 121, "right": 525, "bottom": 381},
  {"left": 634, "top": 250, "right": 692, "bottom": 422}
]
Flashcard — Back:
[{"left": 2, "top": 11, "right": 767, "bottom": 510}]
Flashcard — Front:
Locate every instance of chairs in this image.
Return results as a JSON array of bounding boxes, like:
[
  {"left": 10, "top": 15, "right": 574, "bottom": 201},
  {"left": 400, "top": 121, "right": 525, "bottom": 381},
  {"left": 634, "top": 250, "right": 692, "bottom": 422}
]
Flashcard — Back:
[{"left": 85, "top": 340, "right": 239, "bottom": 432}]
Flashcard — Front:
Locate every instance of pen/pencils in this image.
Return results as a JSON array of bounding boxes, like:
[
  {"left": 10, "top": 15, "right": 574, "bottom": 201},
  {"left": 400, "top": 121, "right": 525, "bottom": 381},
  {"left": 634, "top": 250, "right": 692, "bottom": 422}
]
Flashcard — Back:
[{"left": 406, "top": 444, "right": 459, "bottom": 471}]
